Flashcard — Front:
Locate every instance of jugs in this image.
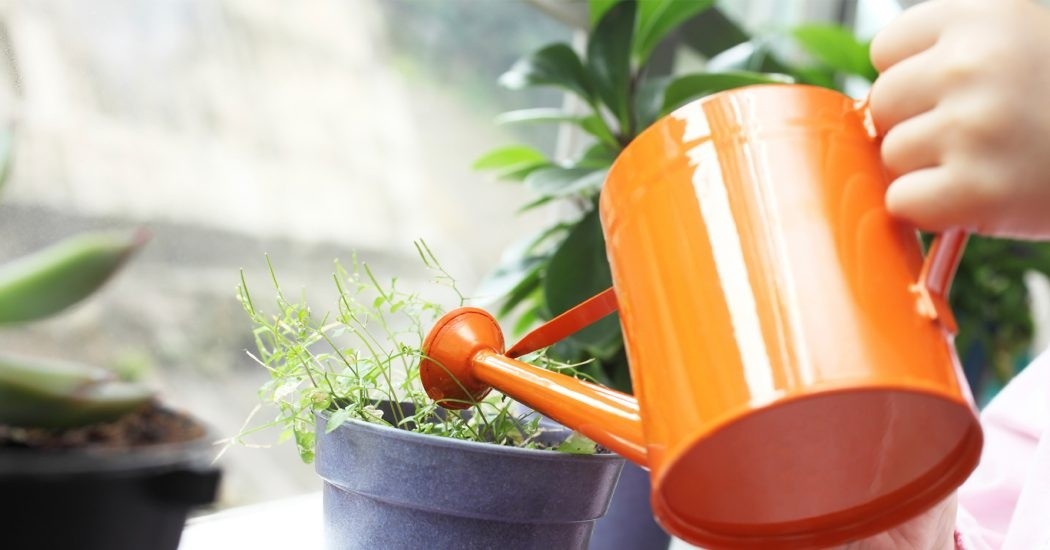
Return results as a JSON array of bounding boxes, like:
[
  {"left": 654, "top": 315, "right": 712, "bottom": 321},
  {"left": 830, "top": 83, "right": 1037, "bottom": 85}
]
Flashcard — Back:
[{"left": 416, "top": 83, "right": 984, "bottom": 550}]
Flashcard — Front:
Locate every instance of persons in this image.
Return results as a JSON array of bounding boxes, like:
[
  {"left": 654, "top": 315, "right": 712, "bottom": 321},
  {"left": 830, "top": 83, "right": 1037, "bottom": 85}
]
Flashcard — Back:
[{"left": 869, "top": 0, "right": 1050, "bottom": 243}]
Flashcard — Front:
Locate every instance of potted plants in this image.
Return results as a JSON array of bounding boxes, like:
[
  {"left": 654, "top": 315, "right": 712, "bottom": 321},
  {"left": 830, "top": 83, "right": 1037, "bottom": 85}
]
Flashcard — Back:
[{"left": 238, "top": 0, "right": 844, "bottom": 550}]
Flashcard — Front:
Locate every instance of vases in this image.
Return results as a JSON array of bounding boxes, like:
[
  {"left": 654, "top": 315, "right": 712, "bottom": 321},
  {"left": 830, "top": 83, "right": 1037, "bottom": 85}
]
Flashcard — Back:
[{"left": 0, "top": 408, "right": 224, "bottom": 550}]
